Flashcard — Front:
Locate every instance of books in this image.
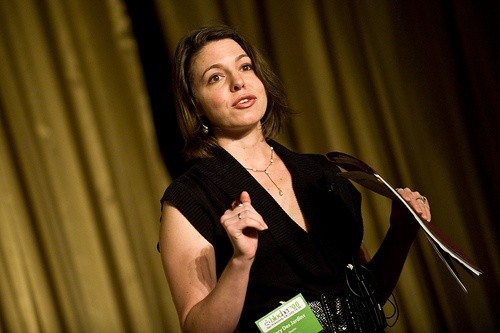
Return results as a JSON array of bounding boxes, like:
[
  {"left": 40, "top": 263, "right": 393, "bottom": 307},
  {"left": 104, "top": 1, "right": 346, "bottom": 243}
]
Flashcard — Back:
[{"left": 325, "top": 150, "right": 483, "bottom": 295}]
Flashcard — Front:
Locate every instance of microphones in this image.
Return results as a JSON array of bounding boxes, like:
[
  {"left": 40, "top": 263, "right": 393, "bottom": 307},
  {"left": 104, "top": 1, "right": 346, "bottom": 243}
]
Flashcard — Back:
[{"left": 202, "top": 124, "right": 209, "bottom": 134}]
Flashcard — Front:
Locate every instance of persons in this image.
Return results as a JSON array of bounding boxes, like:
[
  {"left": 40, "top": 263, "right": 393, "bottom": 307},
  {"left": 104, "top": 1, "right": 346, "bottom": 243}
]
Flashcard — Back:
[{"left": 156, "top": 25, "right": 431, "bottom": 333}]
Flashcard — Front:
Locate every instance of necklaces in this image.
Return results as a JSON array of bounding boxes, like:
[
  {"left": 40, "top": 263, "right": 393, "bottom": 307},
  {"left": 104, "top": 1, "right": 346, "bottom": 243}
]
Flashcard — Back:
[{"left": 245, "top": 147, "right": 284, "bottom": 196}]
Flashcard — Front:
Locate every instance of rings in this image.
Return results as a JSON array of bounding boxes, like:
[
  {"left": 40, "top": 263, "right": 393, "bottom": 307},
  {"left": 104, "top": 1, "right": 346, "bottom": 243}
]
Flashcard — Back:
[
  {"left": 231, "top": 201, "right": 243, "bottom": 211},
  {"left": 237, "top": 213, "right": 242, "bottom": 221},
  {"left": 416, "top": 196, "right": 426, "bottom": 203}
]
